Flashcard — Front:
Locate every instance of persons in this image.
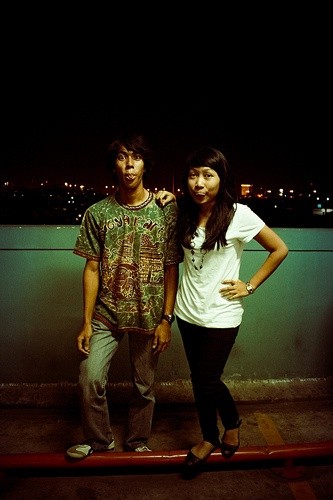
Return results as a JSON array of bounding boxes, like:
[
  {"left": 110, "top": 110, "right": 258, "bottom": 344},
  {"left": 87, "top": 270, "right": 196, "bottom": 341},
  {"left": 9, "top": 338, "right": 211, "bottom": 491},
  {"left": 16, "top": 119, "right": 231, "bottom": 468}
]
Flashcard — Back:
[
  {"left": 155, "top": 147, "right": 290, "bottom": 468},
  {"left": 65, "top": 130, "right": 183, "bottom": 458}
]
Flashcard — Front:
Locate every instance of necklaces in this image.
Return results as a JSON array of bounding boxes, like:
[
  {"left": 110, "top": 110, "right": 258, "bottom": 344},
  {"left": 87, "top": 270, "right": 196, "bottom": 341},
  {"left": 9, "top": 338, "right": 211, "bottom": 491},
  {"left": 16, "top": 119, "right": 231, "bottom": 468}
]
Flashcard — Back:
[{"left": 191, "top": 232, "right": 206, "bottom": 270}]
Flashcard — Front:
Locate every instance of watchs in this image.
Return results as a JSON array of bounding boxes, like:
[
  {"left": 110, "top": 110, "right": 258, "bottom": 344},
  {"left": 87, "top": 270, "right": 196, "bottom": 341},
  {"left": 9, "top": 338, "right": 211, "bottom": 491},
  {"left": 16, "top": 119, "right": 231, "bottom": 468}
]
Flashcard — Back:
[
  {"left": 245, "top": 281, "right": 254, "bottom": 295},
  {"left": 163, "top": 314, "right": 175, "bottom": 323}
]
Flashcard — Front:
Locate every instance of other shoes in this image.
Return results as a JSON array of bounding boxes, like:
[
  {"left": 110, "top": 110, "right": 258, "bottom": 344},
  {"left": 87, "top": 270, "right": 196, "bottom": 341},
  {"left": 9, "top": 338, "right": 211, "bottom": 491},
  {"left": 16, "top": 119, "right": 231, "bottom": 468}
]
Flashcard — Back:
[
  {"left": 220, "top": 419, "right": 243, "bottom": 459},
  {"left": 184, "top": 438, "right": 220, "bottom": 466},
  {"left": 67, "top": 440, "right": 114, "bottom": 458},
  {"left": 130, "top": 441, "right": 152, "bottom": 452}
]
